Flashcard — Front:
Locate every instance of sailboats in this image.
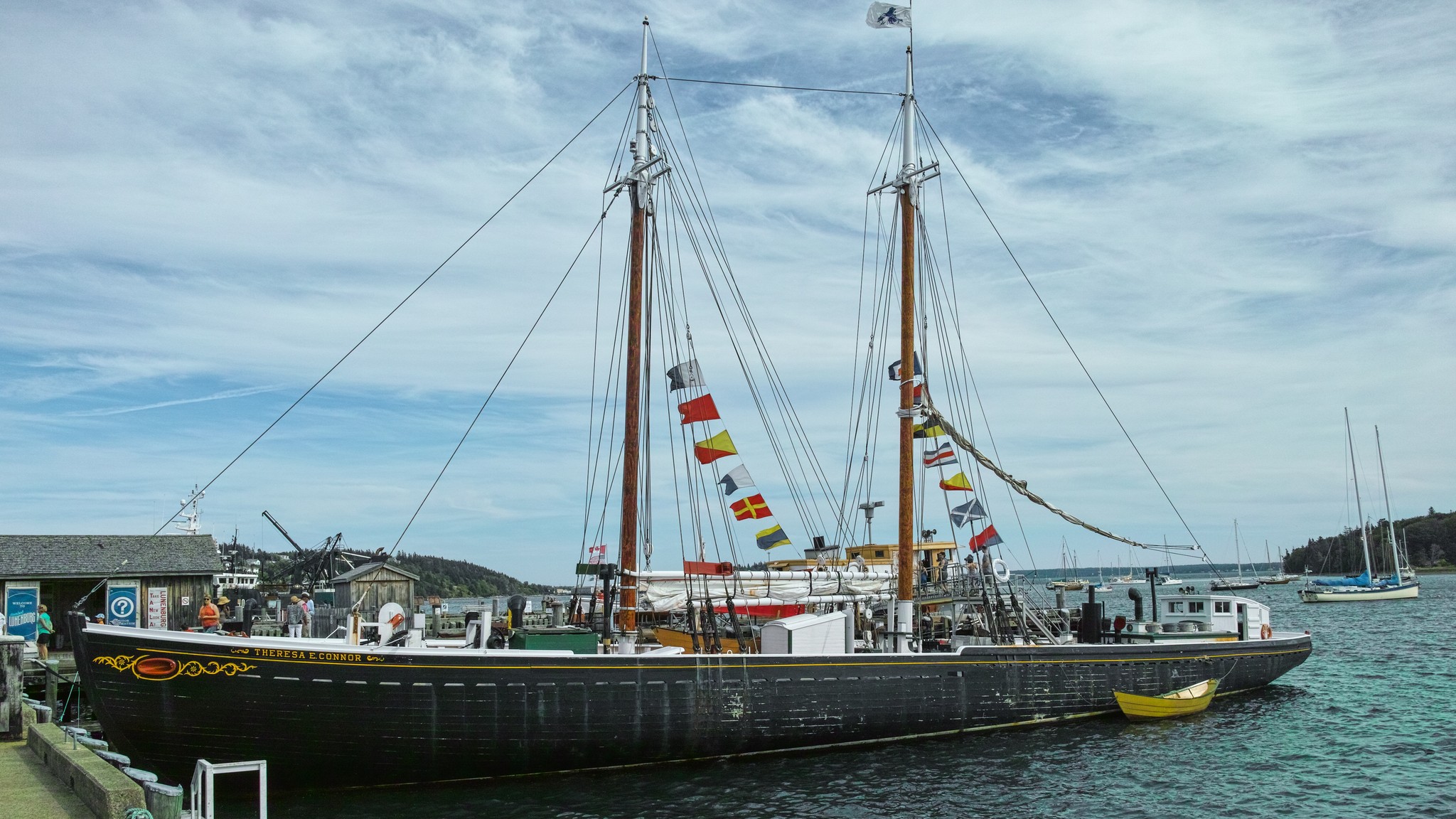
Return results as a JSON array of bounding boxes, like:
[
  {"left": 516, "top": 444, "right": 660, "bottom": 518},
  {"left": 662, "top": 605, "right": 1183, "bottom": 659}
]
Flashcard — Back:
[
  {"left": 1108, "top": 536, "right": 1149, "bottom": 585},
  {"left": 1209, "top": 518, "right": 1260, "bottom": 591},
  {"left": 1045, "top": 535, "right": 1090, "bottom": 591},
  {"left": 1084, "top": 550, "right": 1113, "bottom": 593},
  {"left": 1154, "top": 534, "right": 1182, "bottom": 585},
  {"left": 1255, "top": 540, "right": 1304, "bottom": 584},
  {"left": 1297, "top": 406, "right": 1422, "bottom": 602},
  {"left": 65, "top": 1, "right": 1314, "bottom": 801}
]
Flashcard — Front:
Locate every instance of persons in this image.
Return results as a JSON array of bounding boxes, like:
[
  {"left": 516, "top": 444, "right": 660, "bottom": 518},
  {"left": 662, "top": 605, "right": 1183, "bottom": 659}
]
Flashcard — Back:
[
  {"left": 963, "top": 557, "right": 978, "bottom": 594},
  {"left": 0, "top": 612, "right": 7, "bottom": 636},
  {"left": 37, "top": 604, "right": 55, "bottom": 660},
  {"left": 198, "top": 594, "right": 220, "bottom": 634},
  {"left": 95, "top": 613, "right": 105, "bottom": 624},
  {"left": 598, "top": 589, "right": 604, "bottom": 600},
  {"left": 917, "top": 561, "right": 928, "bottom": 597},
  {"left": 284, "top": 596, "right": 308, "bottom": 638},
  {"left": 181, "top": 623, "right": 194, "bottom": 632},
  {"left": 936, "top": 551, "right": 949, "bottom": 596},
  {"left": 217, "top": 596, "right": 231, "bottom": 619},
  {"left": 979, "top": 545, "right": 994, "bottom": 595},
  {"left": 301, "top": 592, "right": 315, "bottom": 638},
  {"left": 543, "top": 596, "right": 554, "bottom": 608},
  {"left": 85, "top": 616, "right": 90, "bottom": 623}
]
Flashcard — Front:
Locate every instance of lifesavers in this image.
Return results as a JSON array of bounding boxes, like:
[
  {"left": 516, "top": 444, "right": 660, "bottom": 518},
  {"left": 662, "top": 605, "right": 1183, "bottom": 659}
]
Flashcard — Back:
[
  {"left": 1261, "top": 623, "right": 1273, "bottom": 641},
  {"left": 991, "top": 558, "right": 1010, "bottom": 584}
]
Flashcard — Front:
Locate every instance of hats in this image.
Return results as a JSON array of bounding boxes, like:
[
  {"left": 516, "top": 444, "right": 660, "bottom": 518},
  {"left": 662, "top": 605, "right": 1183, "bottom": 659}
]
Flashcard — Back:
[
  {"left": 291, "top": 596, "right": 301, "bottom": 603},
  {"left": 550, "top": 596, "right": 552, "bottom": 598},
  {"left": 217, "top": 597, "right": 230, "bottom": 605},
  {"left": 94, "top": 613, "right": 105, "bottom": 619},
  {"left": 85, "top": 616, "right": 90, "bottom": 621}
]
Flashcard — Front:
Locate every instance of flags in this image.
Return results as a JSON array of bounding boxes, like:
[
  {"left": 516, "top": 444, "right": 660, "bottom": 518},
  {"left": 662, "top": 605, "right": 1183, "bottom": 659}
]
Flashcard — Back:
[
  {"left": 887, "top": 351, "right": 988, "bottom": 530},
  {"left": 590, "top": 554, "right": 606, "bottom": 564},
  {"left": 756, "top": 524, "right": 792, "bottom": 551},
  {"left": 717, "top": 464, "right": 755, "bottom": 496},
  {"left": 666, "top": 359, "right": 738, "bottom": 465},
  {"left": 969, "top": 524, "right": 1003, "bottom": 554},
  {"left": 589, "top": 545, "right": 606, "bottom": 554},
  {"left": 587, "top": 574, "right": 595, "bottom": 582},
  {"left": 729, "top": 493, "right": 773, "bottom": 521}
]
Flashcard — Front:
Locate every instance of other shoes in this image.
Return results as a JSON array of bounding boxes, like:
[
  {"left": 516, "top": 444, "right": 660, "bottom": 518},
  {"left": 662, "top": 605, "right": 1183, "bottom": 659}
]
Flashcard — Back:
[{"left": 943, "top": 591, "right": 949, "bottom": 595}]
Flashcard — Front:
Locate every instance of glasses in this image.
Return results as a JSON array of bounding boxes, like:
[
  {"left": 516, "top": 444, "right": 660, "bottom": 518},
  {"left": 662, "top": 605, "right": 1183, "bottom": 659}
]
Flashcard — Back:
[
  {"left": 37, "top": 608, "right": 40, "bottom": 610},
  {"left": 204, "top": 598, "right": 210, "bottom": 600}
]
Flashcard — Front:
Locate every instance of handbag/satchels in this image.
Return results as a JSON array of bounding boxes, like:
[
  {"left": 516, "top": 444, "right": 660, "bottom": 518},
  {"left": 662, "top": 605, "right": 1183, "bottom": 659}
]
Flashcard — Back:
[
  {"left": 217, "top": 623, "right": 222, "bottom": 630},
  {"left": 302, "top": 625, "right": 309, "bottom": 637},
  {"left": 283, "top": 622, "right": 289, "bottom": 633}
]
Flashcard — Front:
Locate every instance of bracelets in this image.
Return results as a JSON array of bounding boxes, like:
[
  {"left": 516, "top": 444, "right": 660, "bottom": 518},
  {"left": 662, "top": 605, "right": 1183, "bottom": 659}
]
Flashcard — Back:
[{"left": 225, "top": 609, "right": 227, "bottom": 610}]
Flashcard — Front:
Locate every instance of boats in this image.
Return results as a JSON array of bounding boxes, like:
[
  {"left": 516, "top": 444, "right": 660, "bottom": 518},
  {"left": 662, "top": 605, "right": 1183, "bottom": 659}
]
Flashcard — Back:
[
  {"left": 570, "top": 594, "right": 654, "bottom": 615},
  {"left": 1110, "top": 677, "right": 1221, "bottom": 724},
  {"left": 650, "top": 625, "right": 761, "bottom": 655}
]
